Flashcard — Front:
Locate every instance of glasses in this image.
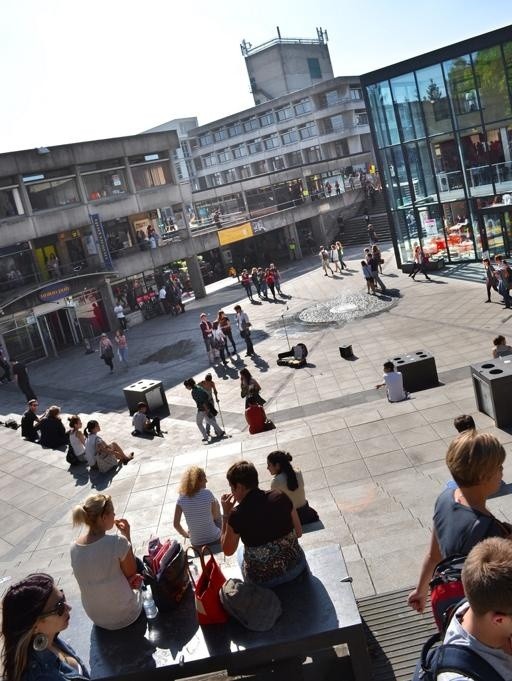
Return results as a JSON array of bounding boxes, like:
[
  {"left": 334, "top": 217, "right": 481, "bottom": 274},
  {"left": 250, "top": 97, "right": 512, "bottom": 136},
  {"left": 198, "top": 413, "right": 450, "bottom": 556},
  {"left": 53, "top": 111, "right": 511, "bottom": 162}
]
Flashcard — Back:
[
  {"left": 92, "top": 492, "right": 112, "bottom": 519},
  {"left": 35, "top": 589, "right": 66, "bottom": 617}
]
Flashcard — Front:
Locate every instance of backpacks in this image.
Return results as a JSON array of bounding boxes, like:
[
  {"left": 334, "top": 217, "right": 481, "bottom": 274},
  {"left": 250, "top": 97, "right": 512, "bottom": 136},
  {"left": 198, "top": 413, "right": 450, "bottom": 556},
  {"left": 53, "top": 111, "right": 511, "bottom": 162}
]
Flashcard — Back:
[
  {"left": 430, "top": 517, "right": 495, "bottom": 631},
  {"left": 413, "top": 597, "right": 506, "bottom": 681},
  {"left": 219, "top": 577, "right": 280, "bottom": 632}
]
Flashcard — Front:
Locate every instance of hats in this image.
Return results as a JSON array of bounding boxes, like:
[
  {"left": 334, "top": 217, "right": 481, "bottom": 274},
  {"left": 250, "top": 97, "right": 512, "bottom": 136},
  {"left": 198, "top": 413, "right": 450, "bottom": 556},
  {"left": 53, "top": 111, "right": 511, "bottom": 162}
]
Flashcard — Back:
[{"left": 3, "top": 571, "right": 54, "bottom": 638}]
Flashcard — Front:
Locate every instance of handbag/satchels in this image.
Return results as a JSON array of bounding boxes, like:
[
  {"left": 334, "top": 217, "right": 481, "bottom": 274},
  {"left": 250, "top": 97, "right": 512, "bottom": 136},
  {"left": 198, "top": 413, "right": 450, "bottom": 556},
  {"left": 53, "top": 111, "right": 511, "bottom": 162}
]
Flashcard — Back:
[
  {"left": 380, "top": 258, "right": 384, "bottom": 264},
  {"left": 66, "top": 447, "right": 76, "bottom": 464},
  {"left": 240, "top": 328, "right": 251, "bottom": 339},
  {"left": 320, "top": 250, "right": 330, "bottom": 260},
  {"left": 263, "top": 418, "right": 276, "bottom": 430},
  {"left": 486, "top": 278, "right": 500, "bottom": 286},
  {"left": 212, "top": 337, "right": 226, "bottom": 350},
  {"left": 143, "top": 538, "right": 193, "bottom": 609},
  {"left": 185, "top": 544, "right": 227, "bottom": 626}
]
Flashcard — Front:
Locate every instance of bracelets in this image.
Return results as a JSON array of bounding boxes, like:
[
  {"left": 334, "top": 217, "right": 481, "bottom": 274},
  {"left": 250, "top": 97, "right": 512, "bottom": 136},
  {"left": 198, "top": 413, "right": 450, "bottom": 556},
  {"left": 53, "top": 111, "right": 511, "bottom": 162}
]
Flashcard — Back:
[{"left": 223, "top": 514, "right": 230, "bottom": 519}]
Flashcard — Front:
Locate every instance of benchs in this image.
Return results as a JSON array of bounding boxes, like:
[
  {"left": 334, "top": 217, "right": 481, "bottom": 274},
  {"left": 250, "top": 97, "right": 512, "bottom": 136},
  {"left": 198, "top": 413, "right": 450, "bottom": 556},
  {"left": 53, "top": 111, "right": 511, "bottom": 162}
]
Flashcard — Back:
[{"left": 56, "top": 544, "right": 377, "bottom": 680}]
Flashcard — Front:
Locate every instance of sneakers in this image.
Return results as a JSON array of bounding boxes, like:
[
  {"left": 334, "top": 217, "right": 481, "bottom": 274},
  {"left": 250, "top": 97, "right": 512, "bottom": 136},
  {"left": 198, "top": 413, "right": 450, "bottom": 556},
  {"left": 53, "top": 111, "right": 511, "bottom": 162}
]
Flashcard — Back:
[
  {"left": 121, "top": 451, "right": 134, "bottom": 465},
  {"left": 484, "top": 295, "right": 511, "bottom": 310},
  {"left": 408, "top": 273, "right": 431, "bottom": 282},
  {"left": 155, "top": 432, "right": 164, "bottom": 438},
  {"left": 208, "top": 350, "right": 258, "bottom": 363},
  {"left": 200, "top": 430, "right": 227, "bottom": 442}
]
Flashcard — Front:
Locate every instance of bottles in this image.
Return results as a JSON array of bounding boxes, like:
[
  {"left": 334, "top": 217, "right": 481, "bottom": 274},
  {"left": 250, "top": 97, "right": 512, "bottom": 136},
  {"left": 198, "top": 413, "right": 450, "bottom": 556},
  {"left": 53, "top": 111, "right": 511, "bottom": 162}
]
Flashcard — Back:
[
  {"left": 141, "top": 586, "right": 158, "bottom": 619},
  {"left": 186, "top": 559, "right": 200, "bottom": 591}
]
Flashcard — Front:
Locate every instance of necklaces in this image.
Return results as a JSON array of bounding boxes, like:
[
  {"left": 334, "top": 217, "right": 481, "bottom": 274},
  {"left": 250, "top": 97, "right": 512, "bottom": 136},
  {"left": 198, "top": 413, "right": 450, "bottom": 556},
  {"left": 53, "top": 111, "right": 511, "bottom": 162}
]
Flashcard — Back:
[{"left": 61, "top": 650, "right": 68, "bottom": 664}]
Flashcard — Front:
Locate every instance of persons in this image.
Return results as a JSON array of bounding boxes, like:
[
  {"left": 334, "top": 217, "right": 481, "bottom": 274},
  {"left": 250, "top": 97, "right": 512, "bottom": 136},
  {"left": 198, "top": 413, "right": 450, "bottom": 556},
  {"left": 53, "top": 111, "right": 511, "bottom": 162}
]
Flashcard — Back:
[
  {"left": 69, "top": 494, "right": 152, "bottom": 631},
  {"left": 0, "top": 572, "right": 93, "bottom": 681},
  {"left": 411, "top": 246, "right": 430, "bottom": 280},
  {"left": 407, "top": 429, "right": 512, "bottom": 628},
  {"left": 375, "top": 361, "right": 408, "bottom": 403},
  {"left": 482, "top": 254, "right": 512, "bottom": 309},
  {"left": 454, "top": 414, "right": 476, "bottom": 433},
  {"left": 173, "top": 466, "right": 222, "bottom": 547},
  {"left": 220, "top": 460, "right": 306, "bottom": 587},
  {"left": 414, "top": 535, "right": 512, "bottom": 681},
  {"left": 266, "top": 450, "right": 320, "bottom": 525},
  {"left": 492, "top": 334, "right": 512, "bottom": 358}
]
